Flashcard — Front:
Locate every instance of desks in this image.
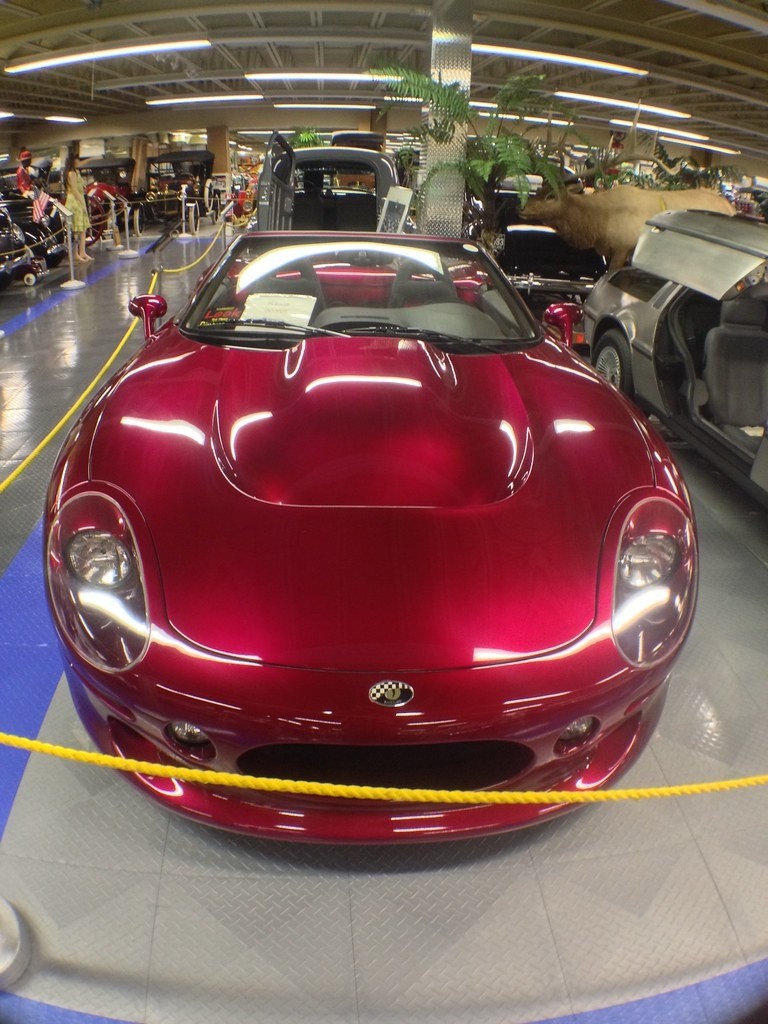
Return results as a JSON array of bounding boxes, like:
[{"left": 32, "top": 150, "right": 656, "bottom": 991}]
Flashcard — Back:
[{"left": 506, "top": 273, "right": 594, "bottom": 309}]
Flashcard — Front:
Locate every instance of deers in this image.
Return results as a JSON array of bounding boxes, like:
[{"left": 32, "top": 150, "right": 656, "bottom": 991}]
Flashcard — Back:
[{"left": 515, "top": 97, "right": 737, "bottom": 271}]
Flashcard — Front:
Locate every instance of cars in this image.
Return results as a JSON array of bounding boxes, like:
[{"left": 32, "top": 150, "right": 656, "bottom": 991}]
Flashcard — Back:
[
  {"left": 246, "top": 130, "right": 419, "bottom": 231},
  {"left": 134, "top": 150, "right": 220, "bottom": 233},
  {"left": 0, "top": 152, "right": 138, "bottom": 289},
  {"left": 466, "top": 221, "right": 609, "bottom": 347}
]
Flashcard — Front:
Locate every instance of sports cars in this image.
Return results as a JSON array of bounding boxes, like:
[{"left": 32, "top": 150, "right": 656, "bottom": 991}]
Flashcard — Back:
[
  {"left": 41, "top": 229, "right": 699, "bottom": 850},
  {"left": 578, "top": 206, "right": 768, "bottom": 509}
]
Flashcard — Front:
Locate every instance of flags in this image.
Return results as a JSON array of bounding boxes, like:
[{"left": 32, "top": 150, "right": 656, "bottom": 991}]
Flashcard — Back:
[{"left": 33, "top": 187, "right": 50, "bottom": 224}]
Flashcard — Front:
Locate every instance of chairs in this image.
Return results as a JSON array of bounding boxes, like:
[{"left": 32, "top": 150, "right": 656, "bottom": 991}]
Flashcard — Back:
[
  {"left": 292, "top": 171, "right": 377, "bottom": 232},
  {"left": 387, "top": 257, "right": 457, "bottom": 308},
  {"left": 256, "top": 255, "right": 326, "bottom": 324},
  {"left": 702, "top": 298, "right": 768, "bottom": 426}
]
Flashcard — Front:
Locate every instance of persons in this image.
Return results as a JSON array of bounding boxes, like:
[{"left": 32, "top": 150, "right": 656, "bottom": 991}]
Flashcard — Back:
[
  {"left": 64, "top": 152, "right": 94, "bottom": 262},
  {"left": 17, "top": 151, "right": 36, "bottom": 199}
]
